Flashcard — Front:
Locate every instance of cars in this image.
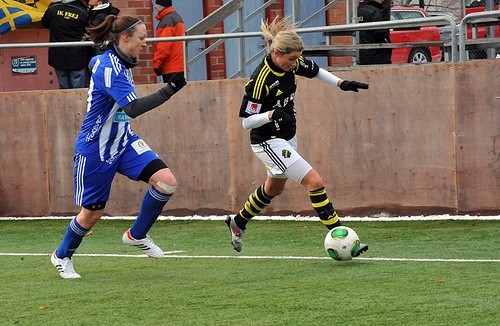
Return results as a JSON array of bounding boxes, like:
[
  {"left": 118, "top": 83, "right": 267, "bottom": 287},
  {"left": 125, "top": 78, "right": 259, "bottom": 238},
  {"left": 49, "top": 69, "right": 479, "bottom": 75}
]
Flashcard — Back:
[{"left": 390, "top": 0, "right": 500, "bottom": 64}]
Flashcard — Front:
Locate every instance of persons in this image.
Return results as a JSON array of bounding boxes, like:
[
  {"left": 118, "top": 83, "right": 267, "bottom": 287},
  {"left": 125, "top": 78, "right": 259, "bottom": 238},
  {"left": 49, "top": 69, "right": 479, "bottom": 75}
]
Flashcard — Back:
[
  {"left": 153, "top": 0, "right": 185, "bottom": 82},
  {"left": 49, "top": 15, "right": 188, "bottom": 277},
  {"left": 225, "top": 14, "right": 369, "bottom": 255},
  {"left": 41, "top": 0, "right": 119, "bottom": 88},
  {"left": 357, "top": 0, "right": 393, "bottom": 64}
]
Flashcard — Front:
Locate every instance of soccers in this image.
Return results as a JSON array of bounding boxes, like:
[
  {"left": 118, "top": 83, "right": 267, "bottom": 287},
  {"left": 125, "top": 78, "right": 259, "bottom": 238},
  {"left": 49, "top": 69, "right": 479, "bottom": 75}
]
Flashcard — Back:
[{"left": 324, "top": 227, "right": 359, "bottom": 261}]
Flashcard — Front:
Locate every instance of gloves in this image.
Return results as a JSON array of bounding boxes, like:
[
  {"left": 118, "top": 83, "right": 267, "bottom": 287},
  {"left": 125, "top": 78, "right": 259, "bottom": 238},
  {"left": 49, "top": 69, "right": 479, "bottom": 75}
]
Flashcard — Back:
[
  {"left": 154, "top": 68, "right": 162, "bottom": 76},
  {"left": 340, "top": 80, "right": 369, "bottom": 92},
  {"left": 272, "top": 108, "right": 295, "bottom": 129},
  {"left": 168, "top": 73, "right": 187, "bottom": 91}
]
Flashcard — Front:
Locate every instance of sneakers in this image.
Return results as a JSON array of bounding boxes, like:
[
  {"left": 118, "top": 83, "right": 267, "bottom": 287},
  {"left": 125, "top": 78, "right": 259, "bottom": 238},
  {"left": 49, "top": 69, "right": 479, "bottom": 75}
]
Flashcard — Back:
[
  {"left": 356, "top": 242, "right": 368, "bottom": 257},
  {"left": 225, "top": 215, "right": 245, "bottom": 252},
  {"left": 122, "top": 228, "right": 164, "bottom": 258},
  {"left": 51, "top": 249, "right": 81, "bottom": 279}
]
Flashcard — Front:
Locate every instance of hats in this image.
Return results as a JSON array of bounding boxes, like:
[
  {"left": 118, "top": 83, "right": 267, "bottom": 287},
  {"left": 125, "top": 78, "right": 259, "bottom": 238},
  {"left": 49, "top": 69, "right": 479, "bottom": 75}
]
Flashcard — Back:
[{"left": 155, "top": 0, "right": 172, "bottom": 7}]
